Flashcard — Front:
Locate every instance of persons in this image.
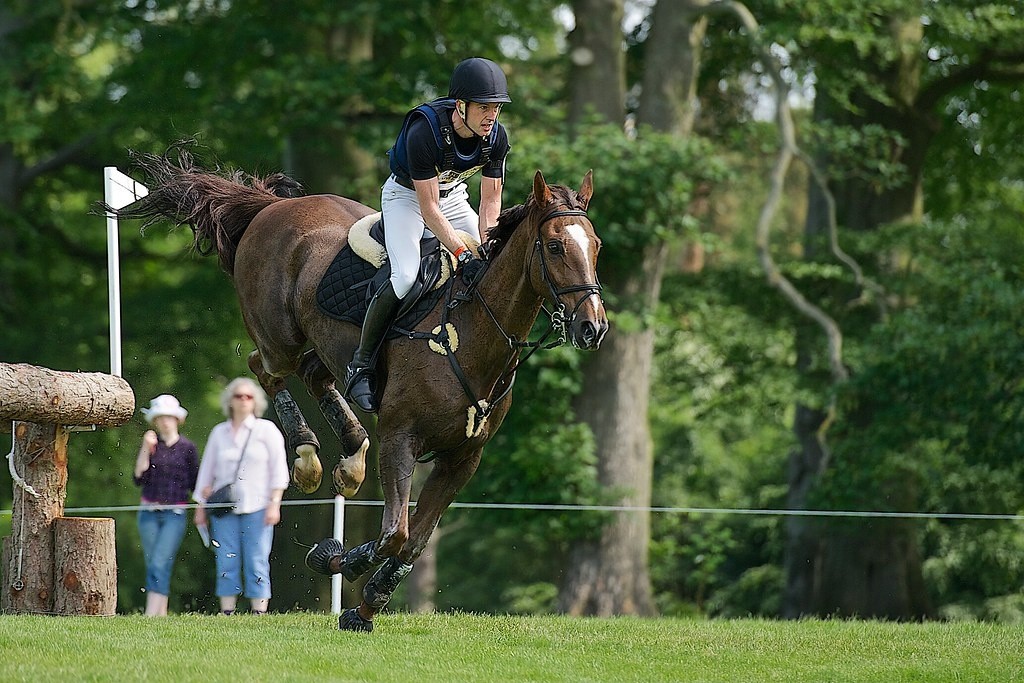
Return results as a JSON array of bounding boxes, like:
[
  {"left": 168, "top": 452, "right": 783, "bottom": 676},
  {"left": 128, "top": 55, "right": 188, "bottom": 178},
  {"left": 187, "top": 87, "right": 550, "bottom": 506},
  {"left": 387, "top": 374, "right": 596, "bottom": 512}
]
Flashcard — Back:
[
  {"left": 133, "top": 393, "right": 211, "bottom": 618},
  {"left": 191, "top": 374, "right": 290, "bottom": 617},
  {"left": 342, "top": 57, "right": 515, "bottom": 413}
]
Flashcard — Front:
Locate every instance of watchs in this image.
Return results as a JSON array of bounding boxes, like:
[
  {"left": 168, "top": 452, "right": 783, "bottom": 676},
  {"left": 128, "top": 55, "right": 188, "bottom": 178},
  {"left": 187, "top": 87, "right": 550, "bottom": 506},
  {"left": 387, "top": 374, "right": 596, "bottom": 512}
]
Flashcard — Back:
[{"left": 456, "top": 248, "right": 472, "bottom": 262}]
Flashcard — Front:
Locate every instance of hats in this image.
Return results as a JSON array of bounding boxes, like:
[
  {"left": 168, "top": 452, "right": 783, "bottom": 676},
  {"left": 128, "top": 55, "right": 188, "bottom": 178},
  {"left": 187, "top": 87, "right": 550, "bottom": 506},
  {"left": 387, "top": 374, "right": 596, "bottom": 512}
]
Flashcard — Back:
[{"left": 144, "top": 394, "right": 188, "bottom": 425}]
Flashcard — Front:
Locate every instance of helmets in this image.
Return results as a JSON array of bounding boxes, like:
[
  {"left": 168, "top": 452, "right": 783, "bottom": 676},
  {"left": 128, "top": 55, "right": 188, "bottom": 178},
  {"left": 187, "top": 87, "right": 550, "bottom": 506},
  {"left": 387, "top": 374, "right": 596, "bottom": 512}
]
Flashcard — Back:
[{"left": 448, "top": 56, "right": 512, "bottom": 103}]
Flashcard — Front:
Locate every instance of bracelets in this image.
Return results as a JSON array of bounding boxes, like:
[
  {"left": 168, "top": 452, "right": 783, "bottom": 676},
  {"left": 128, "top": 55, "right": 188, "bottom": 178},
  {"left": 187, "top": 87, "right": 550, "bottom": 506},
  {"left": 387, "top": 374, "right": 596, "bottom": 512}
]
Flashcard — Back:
[{"left": 454, "top": 244, "right": 468, "bottom": 257}]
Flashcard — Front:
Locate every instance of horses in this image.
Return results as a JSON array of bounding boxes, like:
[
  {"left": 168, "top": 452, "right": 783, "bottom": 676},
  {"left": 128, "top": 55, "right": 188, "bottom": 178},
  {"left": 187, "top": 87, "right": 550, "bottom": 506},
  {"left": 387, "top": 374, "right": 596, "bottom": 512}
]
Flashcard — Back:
[{"left": 85, "top": 118, "right": 610, "bottom": 636}]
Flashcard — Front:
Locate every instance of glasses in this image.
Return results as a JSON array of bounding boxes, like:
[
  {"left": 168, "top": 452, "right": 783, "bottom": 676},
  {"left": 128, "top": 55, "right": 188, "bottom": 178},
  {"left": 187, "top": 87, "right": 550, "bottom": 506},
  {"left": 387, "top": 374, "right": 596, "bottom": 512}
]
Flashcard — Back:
[{"left": 234, "top": 394, "right": 254, "bottom": 399}]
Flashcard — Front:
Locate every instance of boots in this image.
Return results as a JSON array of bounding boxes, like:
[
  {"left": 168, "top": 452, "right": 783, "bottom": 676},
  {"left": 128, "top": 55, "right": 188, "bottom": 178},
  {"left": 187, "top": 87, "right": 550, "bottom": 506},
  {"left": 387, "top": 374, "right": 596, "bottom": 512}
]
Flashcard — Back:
[{"left": 345, "top": 279, "right": 405, "bottom": 413}]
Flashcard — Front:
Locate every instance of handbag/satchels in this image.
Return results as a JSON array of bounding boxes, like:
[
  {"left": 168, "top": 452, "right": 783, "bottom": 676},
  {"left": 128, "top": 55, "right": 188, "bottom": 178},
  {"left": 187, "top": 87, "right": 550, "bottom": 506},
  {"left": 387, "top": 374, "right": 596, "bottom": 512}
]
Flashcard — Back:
[{"left": 205, "top": 483, "right": 237, "bottom": 517}]
